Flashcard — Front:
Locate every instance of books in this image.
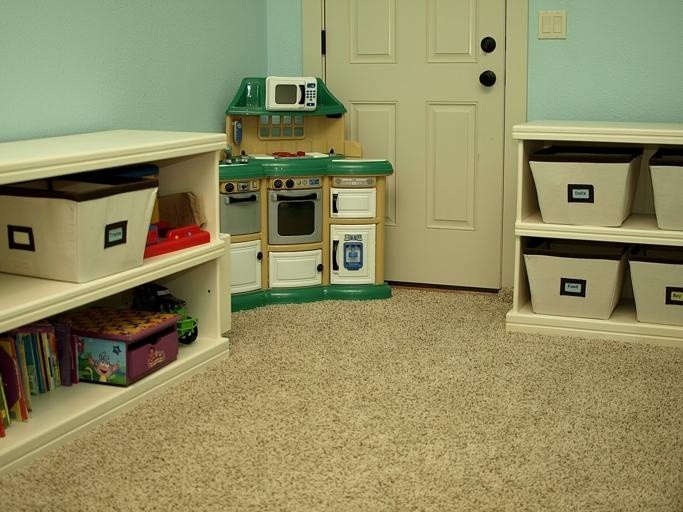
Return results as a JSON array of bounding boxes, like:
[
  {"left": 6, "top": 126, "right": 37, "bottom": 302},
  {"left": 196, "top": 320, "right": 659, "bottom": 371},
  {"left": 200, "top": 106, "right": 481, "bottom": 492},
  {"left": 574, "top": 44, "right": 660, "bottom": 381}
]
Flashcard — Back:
[{"left": 0, "top": 320, "right": 79, "bottom": 437}]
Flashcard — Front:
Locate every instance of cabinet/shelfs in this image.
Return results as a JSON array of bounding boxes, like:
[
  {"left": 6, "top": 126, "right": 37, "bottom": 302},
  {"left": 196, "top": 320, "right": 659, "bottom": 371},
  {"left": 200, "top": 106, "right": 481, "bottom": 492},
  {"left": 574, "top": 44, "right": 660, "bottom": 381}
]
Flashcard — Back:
[
  {"left": 504, "top": 121, "right": 683, "bottom": 349},
  {"left": 0, "top": 128, "right": 230, "bottom": 479}
]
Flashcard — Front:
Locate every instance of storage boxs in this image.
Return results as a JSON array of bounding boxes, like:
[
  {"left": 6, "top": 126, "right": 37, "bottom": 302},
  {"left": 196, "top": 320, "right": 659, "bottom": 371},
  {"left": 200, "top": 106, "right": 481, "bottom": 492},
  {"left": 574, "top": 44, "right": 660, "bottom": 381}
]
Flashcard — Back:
[
  {"left": 522, "top": 147, "right": 683, "bottom": 326},
  {"left": 0, "top": 176, "right": 160, "bottom": 284}
]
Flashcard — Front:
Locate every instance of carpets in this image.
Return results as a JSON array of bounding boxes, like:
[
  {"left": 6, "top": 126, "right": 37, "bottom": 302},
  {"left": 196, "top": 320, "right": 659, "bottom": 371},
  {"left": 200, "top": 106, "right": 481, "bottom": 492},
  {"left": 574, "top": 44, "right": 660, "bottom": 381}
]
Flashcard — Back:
[{"left": 0, "top": 289, "right": 682, "bottom": 512}]
